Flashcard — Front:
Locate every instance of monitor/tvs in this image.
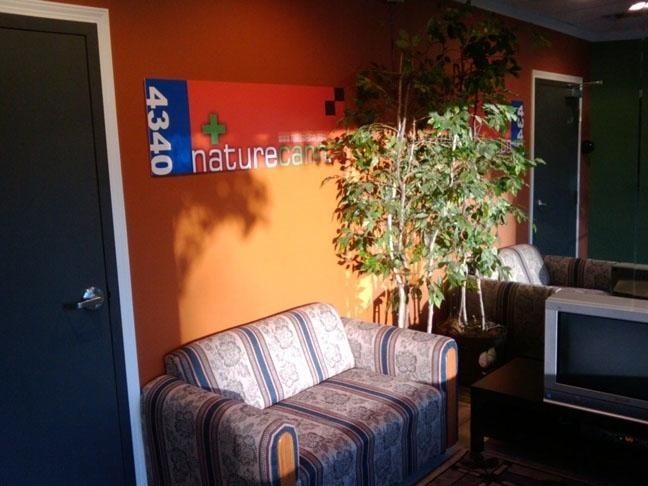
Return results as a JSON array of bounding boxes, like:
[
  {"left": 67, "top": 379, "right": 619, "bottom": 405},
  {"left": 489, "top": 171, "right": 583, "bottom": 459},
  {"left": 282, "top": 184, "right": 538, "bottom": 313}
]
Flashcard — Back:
[{"left": 543, "top": 290, "right": 648, "bottom": 423}]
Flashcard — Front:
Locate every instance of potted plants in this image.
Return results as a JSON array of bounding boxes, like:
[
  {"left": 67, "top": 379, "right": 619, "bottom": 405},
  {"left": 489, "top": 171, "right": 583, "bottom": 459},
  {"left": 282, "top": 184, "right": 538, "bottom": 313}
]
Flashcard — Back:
[{"left": 426, "top": 30, "right": 549, "bottom": 387}]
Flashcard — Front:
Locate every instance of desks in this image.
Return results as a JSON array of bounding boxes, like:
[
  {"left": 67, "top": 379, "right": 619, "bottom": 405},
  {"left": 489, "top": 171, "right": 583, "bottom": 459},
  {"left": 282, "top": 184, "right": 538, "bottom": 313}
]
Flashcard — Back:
[{"left": 471, "top": 354, "right": 648, "bottom": 484}]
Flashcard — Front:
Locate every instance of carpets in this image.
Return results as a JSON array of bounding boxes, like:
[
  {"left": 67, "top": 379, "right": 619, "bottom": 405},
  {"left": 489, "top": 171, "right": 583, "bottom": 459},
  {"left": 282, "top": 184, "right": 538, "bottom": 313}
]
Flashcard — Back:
[{"left": 414, "top": 446, "right": 599, "bottom": 485}]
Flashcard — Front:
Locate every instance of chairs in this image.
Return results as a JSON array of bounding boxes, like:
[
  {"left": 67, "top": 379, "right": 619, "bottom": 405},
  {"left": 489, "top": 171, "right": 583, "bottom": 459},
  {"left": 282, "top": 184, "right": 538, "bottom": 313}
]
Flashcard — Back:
[{"left": 469, "top": 242, "right": 615, "bottom": 363}]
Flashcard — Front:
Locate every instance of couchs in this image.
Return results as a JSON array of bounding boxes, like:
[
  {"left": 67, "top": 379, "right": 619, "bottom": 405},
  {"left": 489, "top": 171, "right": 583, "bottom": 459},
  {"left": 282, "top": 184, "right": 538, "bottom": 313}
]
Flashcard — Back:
[{"left": 141, "top": 302, "right": 461, "bottom": 484}]
